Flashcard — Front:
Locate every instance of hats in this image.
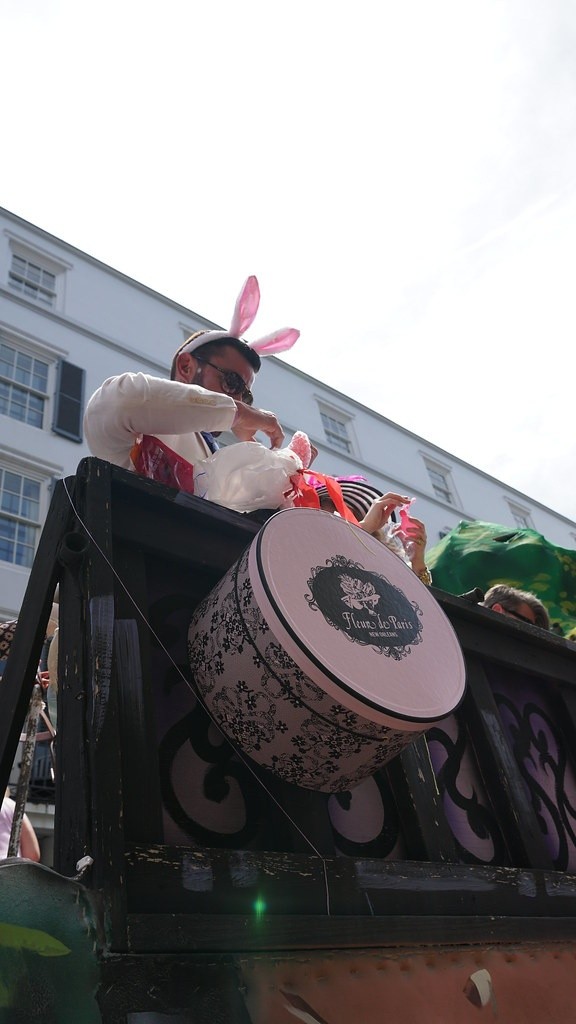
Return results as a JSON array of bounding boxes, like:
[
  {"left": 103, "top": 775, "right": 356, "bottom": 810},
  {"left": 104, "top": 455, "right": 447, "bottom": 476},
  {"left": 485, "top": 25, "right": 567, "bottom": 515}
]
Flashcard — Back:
[{"left": 309, "top": 474, "right": 397, "bottom": 524}]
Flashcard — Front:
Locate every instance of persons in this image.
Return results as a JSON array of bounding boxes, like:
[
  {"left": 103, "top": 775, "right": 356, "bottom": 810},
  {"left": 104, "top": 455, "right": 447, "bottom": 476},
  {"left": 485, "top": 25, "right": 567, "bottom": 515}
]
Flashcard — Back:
[
  {"left": 36, "top": 657, "right": 52, "bottom": 690},
  {"left": 321, "top": 494, "right": 437, "bottom": 587},
  {"left": 82, "top": 331, "right": 286, "bottom": 501},
  {"left": 0, "top": 786, "right": 42, "bottom": 863},
  {"left": 475, "top": 584, "right": 551, "bottom": 632}
]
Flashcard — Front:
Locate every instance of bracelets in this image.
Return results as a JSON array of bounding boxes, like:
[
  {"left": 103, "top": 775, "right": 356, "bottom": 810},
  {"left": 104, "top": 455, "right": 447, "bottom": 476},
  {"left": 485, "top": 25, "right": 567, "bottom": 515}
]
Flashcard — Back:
[{"left": 416, "top": 567, "right": 434, "bottom": 587}]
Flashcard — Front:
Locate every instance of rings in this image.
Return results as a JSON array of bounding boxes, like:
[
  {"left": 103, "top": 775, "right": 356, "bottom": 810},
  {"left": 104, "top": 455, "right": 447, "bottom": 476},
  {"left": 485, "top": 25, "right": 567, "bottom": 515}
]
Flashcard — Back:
[{"left": 416, "top": 538, "right": 423, "bottom": 545}]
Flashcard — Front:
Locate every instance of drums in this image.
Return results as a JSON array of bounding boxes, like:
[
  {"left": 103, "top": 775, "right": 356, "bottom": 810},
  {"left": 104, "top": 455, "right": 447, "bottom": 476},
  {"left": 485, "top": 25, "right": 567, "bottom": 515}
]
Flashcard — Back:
[{"left": 184, "top": 505, "right": 468, "bottom": 797}]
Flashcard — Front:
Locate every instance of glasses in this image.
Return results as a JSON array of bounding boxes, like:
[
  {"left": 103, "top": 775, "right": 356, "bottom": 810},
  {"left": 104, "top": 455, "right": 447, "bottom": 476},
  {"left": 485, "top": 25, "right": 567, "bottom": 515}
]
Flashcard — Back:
[{"left": 192, "top": 355, "right": 254, "bottom": 406}]
